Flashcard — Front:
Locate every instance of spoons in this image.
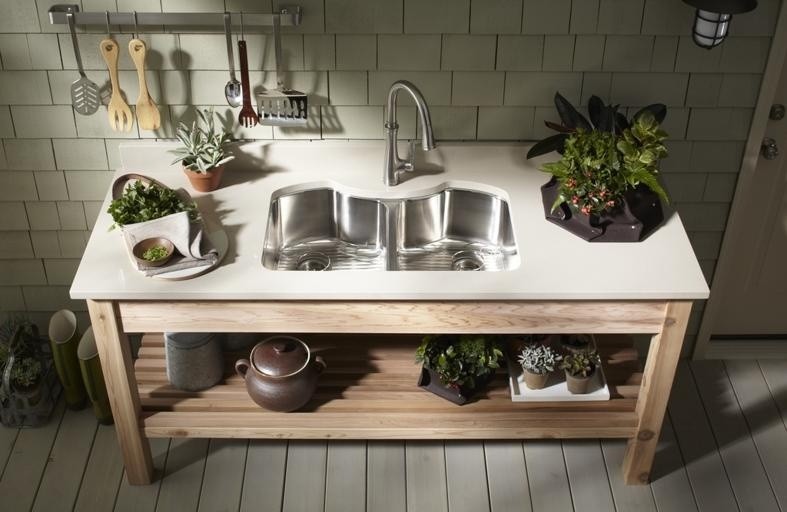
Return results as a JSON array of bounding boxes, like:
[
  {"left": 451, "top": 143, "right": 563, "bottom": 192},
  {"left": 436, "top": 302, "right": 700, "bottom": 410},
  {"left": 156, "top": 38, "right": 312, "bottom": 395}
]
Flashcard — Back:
[{"left": 223, "top": 12, "right": 243, "bottom": 108}]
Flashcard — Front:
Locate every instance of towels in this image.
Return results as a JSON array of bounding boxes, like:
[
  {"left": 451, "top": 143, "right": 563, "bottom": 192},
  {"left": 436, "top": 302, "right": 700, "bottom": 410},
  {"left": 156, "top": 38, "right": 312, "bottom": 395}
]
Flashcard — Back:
[{"left": 117, "top": 210, "right": 219, "bottom": 276}]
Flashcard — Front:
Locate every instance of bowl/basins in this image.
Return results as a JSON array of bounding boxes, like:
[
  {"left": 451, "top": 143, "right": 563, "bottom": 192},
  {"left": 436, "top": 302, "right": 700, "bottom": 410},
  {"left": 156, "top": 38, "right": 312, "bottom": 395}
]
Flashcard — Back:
[{"left": 132, "top": 238, "right": 176, "bottom": 267}]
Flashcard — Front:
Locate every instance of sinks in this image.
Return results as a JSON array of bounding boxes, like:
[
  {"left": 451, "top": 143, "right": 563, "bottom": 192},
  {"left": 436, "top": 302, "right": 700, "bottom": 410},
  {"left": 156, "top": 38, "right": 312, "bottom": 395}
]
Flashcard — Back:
[
  {"left": 391, "top": 181, "right": 519, "bottom": 271},
  {"left": 261, "top": 181, "right": 390, "bottom": 270}
]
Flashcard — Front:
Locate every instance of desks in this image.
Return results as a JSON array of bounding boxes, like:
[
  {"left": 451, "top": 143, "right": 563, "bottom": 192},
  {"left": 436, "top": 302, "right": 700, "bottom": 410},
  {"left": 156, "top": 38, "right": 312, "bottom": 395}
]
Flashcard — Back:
[{"left": 68, "top": 138, "right": 711, "bottom": 485}]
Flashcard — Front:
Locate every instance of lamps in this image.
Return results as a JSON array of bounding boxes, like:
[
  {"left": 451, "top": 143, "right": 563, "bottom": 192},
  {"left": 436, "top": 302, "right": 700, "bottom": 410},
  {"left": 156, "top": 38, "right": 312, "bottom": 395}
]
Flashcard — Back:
[{"left": 683, "top": 1, "right": 758, "bottom": 49}]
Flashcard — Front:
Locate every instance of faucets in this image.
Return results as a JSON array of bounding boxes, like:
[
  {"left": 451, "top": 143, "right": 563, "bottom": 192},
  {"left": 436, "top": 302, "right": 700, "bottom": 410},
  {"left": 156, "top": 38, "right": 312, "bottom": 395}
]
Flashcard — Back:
[{"left": 384, "top": 81, "right": 436, "bottom": 186}]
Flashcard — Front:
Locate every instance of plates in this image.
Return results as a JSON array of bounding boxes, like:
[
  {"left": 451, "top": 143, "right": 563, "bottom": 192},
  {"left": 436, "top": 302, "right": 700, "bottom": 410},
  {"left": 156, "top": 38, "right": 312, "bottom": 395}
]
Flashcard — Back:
[{"left": 155, "top": 216, "right": 229, "bottom": 279}]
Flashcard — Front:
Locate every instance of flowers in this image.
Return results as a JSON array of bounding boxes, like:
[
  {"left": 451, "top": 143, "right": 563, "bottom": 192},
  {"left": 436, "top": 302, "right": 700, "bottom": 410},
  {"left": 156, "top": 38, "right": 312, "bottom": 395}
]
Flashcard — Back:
[{"left": 536, "top": 109, "right": 671, "bottom": 214}]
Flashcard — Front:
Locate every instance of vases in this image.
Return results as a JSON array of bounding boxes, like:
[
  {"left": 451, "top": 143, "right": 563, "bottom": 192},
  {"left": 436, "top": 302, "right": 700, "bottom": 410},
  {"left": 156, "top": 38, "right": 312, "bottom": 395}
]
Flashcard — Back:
[{"left": 539, "top": 175, "right": 665, "bottom": 243}]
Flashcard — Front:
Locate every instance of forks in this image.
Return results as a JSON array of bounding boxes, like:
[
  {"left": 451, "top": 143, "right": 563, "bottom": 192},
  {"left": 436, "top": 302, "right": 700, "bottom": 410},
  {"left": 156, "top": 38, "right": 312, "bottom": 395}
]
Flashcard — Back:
[
  {"left": 238, "top": 40, "right": 258, "bottom": 128},
  {"left": 99, "top": 39, "right": 134, "bottom": 132}
]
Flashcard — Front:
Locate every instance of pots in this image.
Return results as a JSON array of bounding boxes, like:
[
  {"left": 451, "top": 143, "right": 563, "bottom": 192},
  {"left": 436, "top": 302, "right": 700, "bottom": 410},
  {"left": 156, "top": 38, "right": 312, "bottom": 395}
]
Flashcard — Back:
[{"left": 234, "top": 337, "right": 328, "bottom": 413}]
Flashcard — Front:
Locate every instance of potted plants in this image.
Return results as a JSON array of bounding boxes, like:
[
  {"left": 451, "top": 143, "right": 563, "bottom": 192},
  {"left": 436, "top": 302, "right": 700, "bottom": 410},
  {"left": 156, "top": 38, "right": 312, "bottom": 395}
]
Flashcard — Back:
[
  {"left": 558, "top": 334, "right": 599, "bottom": 362},
  {"left": 517, "top": 345, "right": 562, "bottom": 390},
  {"left": 563, "top": 350, "right": 601, "bottom": 393},
  {"left": 0, "top": 315, "right": 62, "bottom": 427},
  {"left": 167, "top": 110, "right": 236, "bottom": 191},
  {"left": 507, "top": 334, "right": 552, "bottom": 355},
  {"left": 415, "top": 336, "right": 503, "bottom": 405}
]
